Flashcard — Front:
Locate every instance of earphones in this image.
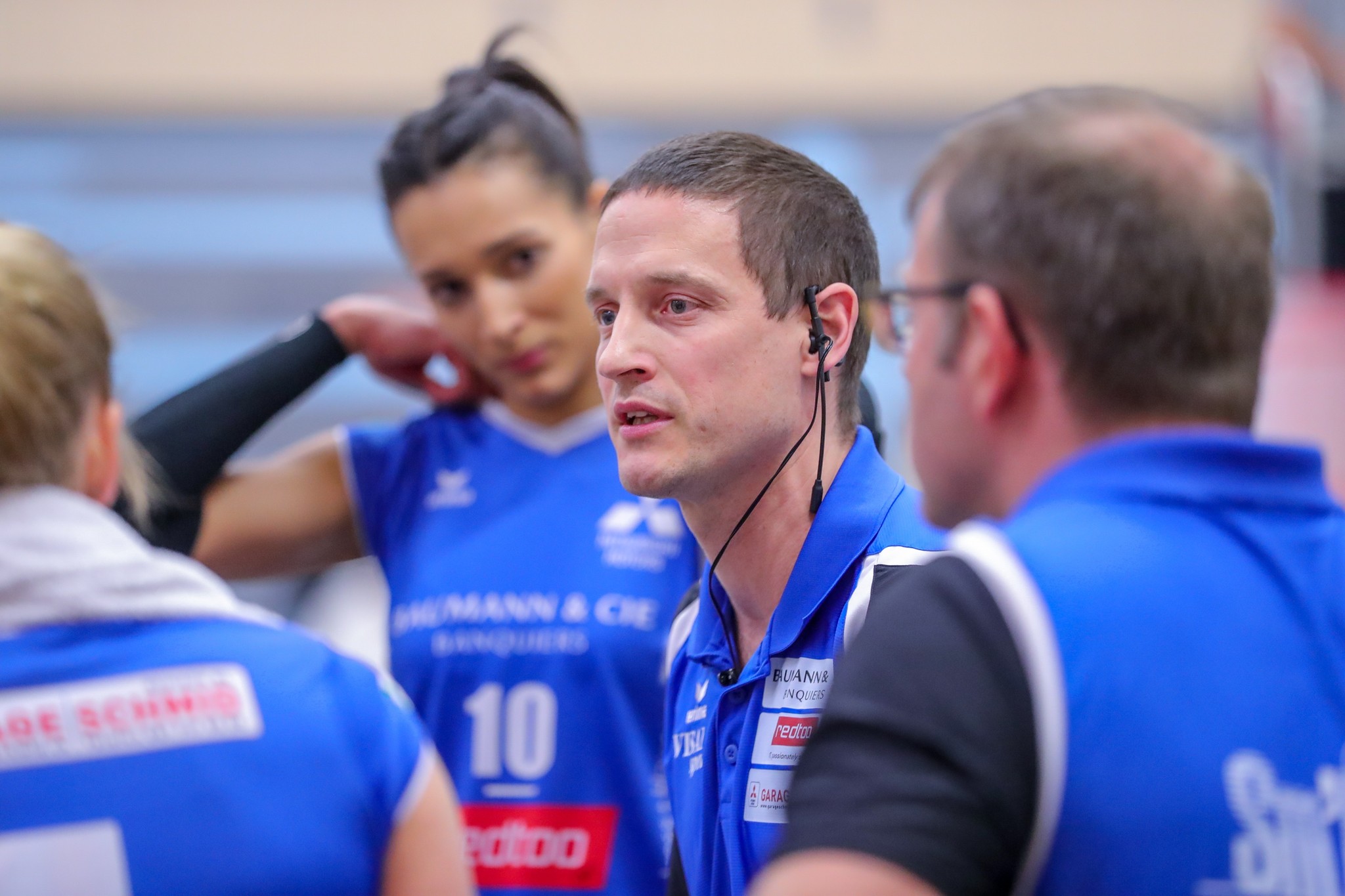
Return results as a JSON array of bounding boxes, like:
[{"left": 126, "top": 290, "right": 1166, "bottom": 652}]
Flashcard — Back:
[{"left": 806, "top": 331, "right": 827, "bottom": 354}]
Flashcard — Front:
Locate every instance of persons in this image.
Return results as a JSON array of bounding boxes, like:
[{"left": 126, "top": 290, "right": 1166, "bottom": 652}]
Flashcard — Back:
[
  {"left": 101, "top": 22, "right": 715, "bottom": 894},
  {"left": 739, "top": 100, "right": 1345, "bottom": 894},
  {"left": 580, "top": 132, "right": 952, "bottom": 894},
  {"left": 0, "top": 216, "right": 480, "bottom": 895}
]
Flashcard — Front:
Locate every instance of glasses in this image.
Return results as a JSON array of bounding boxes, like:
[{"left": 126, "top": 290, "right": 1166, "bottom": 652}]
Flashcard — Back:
[{"left": 868, "top": 277, "right": 1030, "bottom": 359}]
